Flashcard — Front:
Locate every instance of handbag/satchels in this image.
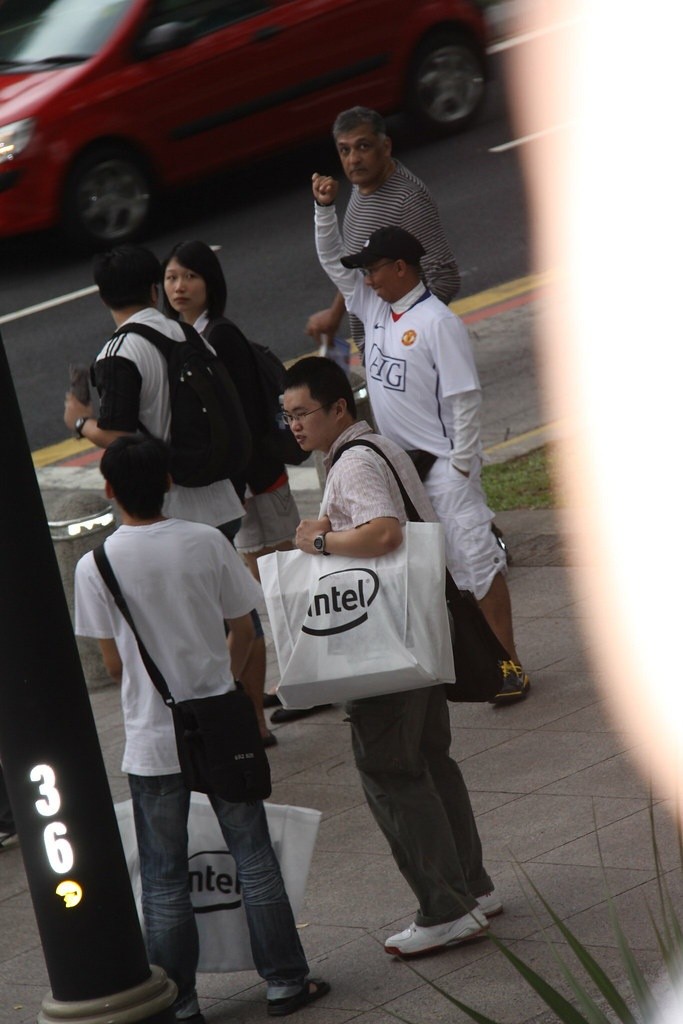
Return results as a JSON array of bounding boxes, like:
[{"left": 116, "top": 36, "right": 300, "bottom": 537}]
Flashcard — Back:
[
  {"left": 113, "top": 789, "right": 322, "bottom": 974},
  {"left": 440, "top": 586, "right": 512, "bottom": 703},
  {"left": 255, "top": 443, "right": 459, "bottom": 709},
  {"left": 171, "top": 686, "right": 272, "bottom": 801}
]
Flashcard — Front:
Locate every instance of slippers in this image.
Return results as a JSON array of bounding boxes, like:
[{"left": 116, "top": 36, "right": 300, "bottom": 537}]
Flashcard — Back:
[{"left": 261, "top": 730, "right": 278, "bottom": 748}]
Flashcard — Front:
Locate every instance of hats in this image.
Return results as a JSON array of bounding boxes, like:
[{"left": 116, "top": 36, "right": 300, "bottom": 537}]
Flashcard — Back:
[{"left": 340, "top": 227, "right": 427, "bottom": 269}]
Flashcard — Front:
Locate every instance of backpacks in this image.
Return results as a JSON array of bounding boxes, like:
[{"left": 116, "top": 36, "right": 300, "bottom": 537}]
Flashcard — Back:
[
  {"left": 202, "top": 319, "right": 314, "bottom": 466},
  {"left": 109, "top": 321, "right": 250, "bottom": 488}
]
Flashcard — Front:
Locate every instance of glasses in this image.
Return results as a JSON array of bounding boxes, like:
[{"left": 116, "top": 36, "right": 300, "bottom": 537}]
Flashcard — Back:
[
  {"left": 359, "top": 258, "right": 402, "bottom": 277},
  {"left": 282, "top": 396, "right": 342, "bottom": 425}
]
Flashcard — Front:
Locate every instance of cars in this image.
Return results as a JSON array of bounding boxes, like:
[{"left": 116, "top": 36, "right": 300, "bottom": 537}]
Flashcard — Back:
[{"left": 0, "top": 1, "right": 489, "bottom": 257}]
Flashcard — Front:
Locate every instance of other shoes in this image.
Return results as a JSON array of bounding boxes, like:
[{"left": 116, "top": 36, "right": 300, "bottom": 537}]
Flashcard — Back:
[
  {"left": 261, "top": 690, "right": 282, "bottom": 707},
  {"left": 271, "top": 698, "right": 331, "bottom": 724}
]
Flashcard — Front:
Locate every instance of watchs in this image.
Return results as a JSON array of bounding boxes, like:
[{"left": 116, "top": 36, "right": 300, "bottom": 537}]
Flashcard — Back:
[
  {"left": 75, "top": 416, "right": 88, "bottom": 440},
  {"left": 313, "top": 530, "right": 331, "bottom": 557}
]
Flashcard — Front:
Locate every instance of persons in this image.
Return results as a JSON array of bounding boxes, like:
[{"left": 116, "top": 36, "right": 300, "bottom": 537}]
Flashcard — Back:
[
  {"left": 277, "top": 354, "right": 500, "bottom": 960},
  {"left": 63, "top": 246, "right": 276, "bottom": 747},
  {"left": 159, "top": 242, "right": 301, "bottom": 585},
  {"left": 305, "top": 104, "right": 462, "bottom": 430},
  {"left": 311, "top": 169, "right": 530, "bottom": 703},
  {"left": 72, "top": 435, "right": 333, "bottom": 1024}
]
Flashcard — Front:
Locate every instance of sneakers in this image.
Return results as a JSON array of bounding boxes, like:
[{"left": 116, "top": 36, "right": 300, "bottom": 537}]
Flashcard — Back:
[
  {"left": 475, "top": 889, "right": 504, "bottom": 916},
  {"left": 491, "top": 655, "right": 530, "bottom": 705},
  {"left": 384, "top": 903, "right": 490, "bottom": 955}
]
